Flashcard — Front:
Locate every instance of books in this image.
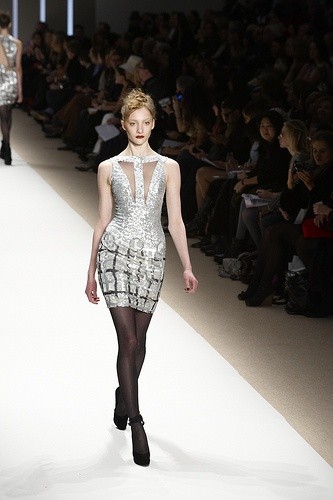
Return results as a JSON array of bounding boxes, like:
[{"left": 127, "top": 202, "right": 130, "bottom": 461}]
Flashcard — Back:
[
  {"left": 94, "top": 120, "right": 121, "bottom": 142},
  {"left": 190, "top": 148, "right": 218, "bottom": 168},
  {"left": 162, "top": 139, "right": 193, "bottom": 152}
]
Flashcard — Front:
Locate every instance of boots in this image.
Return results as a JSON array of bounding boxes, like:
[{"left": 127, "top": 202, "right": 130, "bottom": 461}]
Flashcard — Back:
[
  {"left": 238, "top": 257, "right": 265, "bottom": 300},
  {"left": 245, "top": 257, "right": 280, "bottom": 307}
]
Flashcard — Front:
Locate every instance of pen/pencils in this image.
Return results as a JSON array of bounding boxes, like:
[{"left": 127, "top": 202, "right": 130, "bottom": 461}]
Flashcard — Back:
[{"left": 228, "top": 170, "right": 252, "bottom": 173}]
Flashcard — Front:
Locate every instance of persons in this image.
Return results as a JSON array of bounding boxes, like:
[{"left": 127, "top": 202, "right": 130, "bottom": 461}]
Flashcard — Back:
[
  {"left": 84, "top": 87, "right": 200, "bottom": 467},
  {"left": 0, "top": 13, "right": 25, "bottom": 166},
  {"left": 0, "top": 0, "right": 333, "bottom": 321}
]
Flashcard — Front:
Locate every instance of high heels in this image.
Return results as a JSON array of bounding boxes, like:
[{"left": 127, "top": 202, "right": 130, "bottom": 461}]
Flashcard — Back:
[
  {"left": 113, "top": 387, "right": 128, "bottom": 430},
  {"left": 4, "top": 146, "right": 12, "bottom": 165},
  {"left": 0, "top": 139, "right": 6, "bottom": 159},
  {"left": 129, "top": 415, "right": 150, "bottom": 467}
]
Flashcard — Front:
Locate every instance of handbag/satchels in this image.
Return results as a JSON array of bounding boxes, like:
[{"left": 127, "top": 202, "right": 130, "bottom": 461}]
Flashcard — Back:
[{"left": 285, "top": 270, "right": 312, "bottom": 314}]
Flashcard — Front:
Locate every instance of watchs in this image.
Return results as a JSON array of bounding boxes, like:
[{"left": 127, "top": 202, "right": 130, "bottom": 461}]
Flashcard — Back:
[
  {"left": 241, "top": 176, "right": 247, "bottom": 187},
  {"left": 59, "top": 85, "right": 63, "bottom": 90}
]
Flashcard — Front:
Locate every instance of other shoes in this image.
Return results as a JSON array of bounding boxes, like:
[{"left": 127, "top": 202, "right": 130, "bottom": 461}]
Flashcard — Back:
[
  {"left": 304, "top": 304, "right": 333, "bottom": 318},
  {"left": 15, "top": 98, "right": 98, "bottom": 172},
  {"left": 163, "top": 192, "right": 257, "bottom": 282}
]
